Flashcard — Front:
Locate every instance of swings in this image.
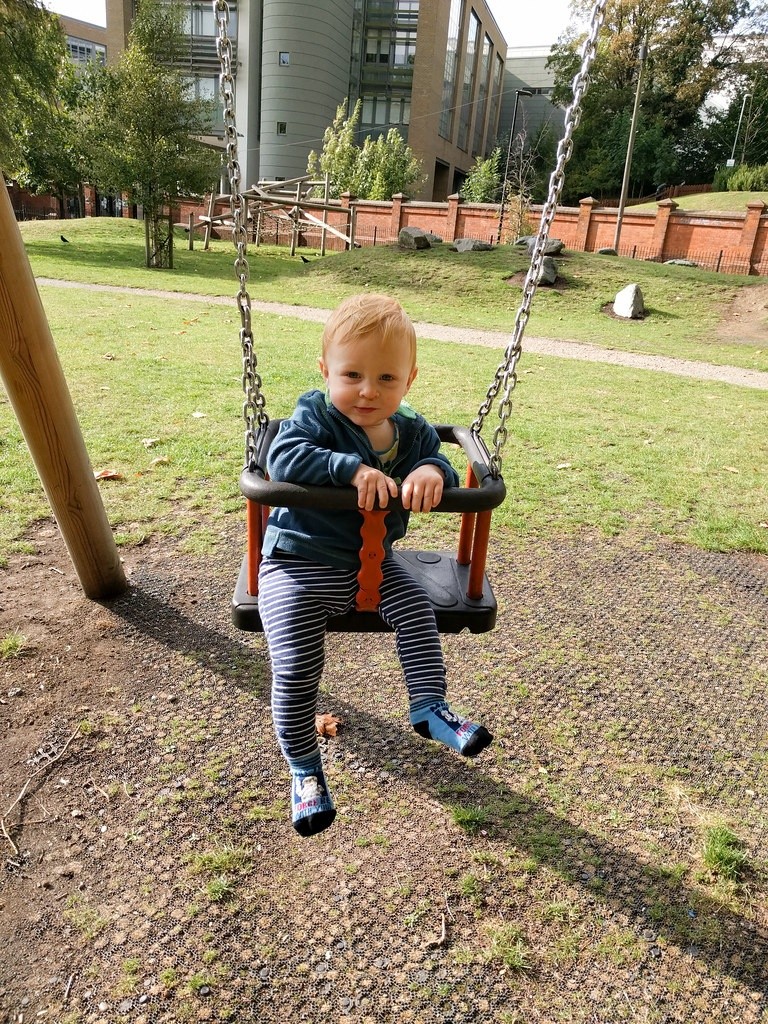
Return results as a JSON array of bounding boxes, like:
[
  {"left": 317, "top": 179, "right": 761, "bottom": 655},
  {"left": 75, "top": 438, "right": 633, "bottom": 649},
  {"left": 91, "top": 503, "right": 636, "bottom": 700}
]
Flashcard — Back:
[{"left": 210, "top": 0, "right": 610, "bottom": 636}]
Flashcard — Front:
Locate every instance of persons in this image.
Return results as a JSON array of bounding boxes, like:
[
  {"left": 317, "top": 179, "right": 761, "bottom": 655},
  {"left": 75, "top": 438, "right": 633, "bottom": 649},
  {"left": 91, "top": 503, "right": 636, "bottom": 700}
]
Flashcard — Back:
[{"left": 259, "top": 294, "right": 494, "bottom": 836}]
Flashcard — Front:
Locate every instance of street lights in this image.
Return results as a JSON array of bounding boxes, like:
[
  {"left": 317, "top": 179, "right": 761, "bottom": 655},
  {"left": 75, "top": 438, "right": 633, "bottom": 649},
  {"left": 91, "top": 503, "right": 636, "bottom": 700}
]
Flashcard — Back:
[
  {"left": 730, "top": 93, "right": 753, "bottom": 167},
  {"left": 496, "top": 89, "right": 532, "bottom": 245}
]
